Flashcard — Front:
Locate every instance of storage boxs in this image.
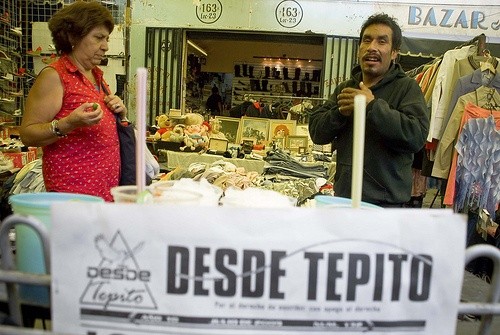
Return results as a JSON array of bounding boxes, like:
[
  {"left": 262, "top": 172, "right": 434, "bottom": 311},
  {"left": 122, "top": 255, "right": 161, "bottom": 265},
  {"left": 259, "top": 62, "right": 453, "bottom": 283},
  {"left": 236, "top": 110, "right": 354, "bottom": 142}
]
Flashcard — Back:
[
  {"left": 3, "top": 147, "right": 38, "bottom": 167},
  {"left": 208, "top": 138, "right": 228, "bottom": 154}
]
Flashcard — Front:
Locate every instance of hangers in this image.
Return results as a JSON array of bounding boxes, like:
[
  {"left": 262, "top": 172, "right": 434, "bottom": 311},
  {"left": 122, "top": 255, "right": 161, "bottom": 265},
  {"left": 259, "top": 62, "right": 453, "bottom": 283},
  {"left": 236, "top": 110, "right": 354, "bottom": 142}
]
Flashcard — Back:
[{"left": 448, "top": 38, "right": 500, "bottom": 134}]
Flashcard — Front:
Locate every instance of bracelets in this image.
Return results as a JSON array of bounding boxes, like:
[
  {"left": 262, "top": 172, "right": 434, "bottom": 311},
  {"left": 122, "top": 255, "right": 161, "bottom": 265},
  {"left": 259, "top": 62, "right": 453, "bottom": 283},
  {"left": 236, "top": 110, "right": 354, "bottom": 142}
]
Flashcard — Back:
[{"left": 50, "top": 119, "right": 65, "bottom": 139}]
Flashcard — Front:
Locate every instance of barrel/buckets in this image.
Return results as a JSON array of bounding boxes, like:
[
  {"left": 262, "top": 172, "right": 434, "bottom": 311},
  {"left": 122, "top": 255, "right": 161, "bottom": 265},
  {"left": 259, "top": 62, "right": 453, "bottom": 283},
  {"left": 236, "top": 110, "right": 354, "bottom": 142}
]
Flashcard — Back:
[
  {"left": 9, "top": 193, "right": 105, "bottom": 303},
  {"left": 110, "top": 179, "right": 383, "bottom": 209}
]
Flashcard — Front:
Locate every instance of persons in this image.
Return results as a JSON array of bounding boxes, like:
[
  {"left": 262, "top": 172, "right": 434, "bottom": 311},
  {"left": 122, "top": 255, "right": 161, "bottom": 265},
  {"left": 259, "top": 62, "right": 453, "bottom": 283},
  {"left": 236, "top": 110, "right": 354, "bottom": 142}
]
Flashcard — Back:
[
  {"left": 19, "top": 1, "right": 126, "bottom": 202},
  {"left": 308, "top": 13, "right": 429, "bottom": 208}
]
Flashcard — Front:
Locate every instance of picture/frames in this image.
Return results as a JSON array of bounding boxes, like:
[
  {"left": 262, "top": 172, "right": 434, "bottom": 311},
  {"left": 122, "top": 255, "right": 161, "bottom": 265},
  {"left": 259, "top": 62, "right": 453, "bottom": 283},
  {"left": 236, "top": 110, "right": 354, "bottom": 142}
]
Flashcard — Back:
[
  {"left": 215, "top": 116, "right": 240, "bottom": 143},
  {"left": 286, "top": 135, "right": 309, "bottom": 150},
  {"left": 242, "top": 116, "right": 270, "bottom": 144},
  {"left": 269, "top": 119, "right": 298, "bottom": 146}
]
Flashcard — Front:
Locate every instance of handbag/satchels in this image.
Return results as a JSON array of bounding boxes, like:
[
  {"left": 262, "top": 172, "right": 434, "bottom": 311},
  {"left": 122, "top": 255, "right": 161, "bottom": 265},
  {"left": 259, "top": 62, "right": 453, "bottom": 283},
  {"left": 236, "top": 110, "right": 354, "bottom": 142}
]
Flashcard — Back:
[{"left": 114, "top": 115, "right": 160, "bottom": 187}]
